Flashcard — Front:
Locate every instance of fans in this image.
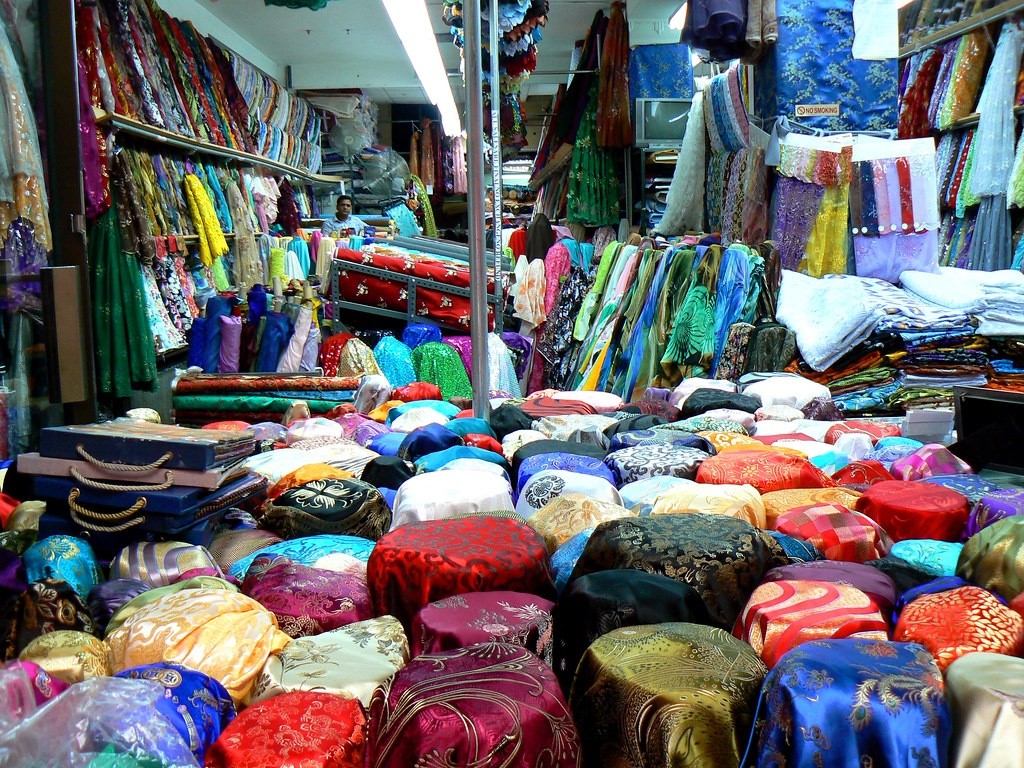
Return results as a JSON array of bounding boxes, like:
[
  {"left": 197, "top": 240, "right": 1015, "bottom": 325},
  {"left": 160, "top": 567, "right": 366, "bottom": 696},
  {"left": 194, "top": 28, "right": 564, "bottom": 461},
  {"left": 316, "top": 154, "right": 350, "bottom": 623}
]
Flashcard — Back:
[
  {"left": 363, "top": 151, "right": 412, "bottom": 198},
  {"left": 329, "top": 119, "right": 367, "bottom": 158}
]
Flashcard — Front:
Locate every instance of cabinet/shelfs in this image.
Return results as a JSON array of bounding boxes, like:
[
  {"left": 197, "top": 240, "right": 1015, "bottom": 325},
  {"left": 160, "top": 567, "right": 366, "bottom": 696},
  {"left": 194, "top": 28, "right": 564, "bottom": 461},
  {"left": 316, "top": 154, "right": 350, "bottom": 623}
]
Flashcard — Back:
[
  {"left": 74, "top": 109, "right": 342, "bottom": 360},
  {"left": 896, "top": 0, "right": 1024, "bottom": 132},
  {"left": 330, "top": 234, "right": 511, "bottom": 350}
]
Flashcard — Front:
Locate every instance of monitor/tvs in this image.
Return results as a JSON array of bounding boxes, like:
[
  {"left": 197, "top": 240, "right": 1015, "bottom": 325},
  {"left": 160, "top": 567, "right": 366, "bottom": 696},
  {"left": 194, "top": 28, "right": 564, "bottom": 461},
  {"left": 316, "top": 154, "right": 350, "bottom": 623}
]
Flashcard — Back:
[{"left": 633, "top": 97, "right": 694, "bottom": 152}]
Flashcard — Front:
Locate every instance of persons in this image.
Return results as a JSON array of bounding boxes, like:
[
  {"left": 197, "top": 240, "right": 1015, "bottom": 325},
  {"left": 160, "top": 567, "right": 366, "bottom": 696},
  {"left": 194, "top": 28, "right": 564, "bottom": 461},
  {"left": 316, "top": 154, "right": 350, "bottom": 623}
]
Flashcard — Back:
[{"left": 322, "top": 195, "right": 370, "bottom": 235}]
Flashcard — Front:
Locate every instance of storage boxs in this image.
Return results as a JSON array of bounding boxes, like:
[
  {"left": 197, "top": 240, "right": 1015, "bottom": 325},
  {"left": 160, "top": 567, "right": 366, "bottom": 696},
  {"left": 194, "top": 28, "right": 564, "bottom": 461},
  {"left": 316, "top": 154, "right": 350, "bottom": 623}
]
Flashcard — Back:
[{"left": 53, "top": 266, "right": 89, "bottom": 403}]
